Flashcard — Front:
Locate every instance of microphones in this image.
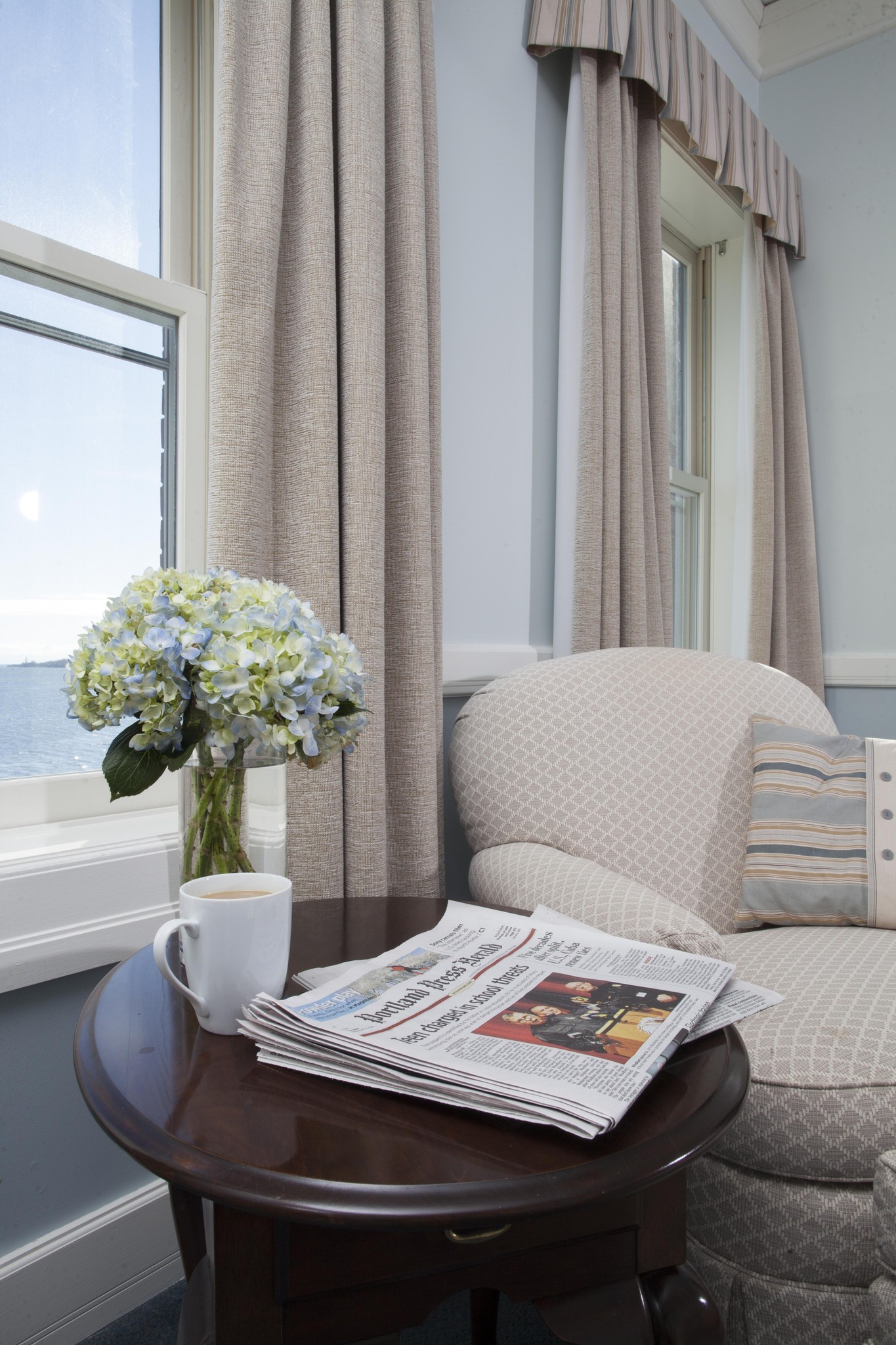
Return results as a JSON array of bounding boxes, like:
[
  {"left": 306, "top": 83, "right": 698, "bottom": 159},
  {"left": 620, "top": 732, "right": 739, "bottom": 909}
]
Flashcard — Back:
[
  {"left": 576, "top": 996, "right": 624, "bottom": 1021},
  {"left": 567, "top": 1032, "right": 598, "bottom": 1040}
]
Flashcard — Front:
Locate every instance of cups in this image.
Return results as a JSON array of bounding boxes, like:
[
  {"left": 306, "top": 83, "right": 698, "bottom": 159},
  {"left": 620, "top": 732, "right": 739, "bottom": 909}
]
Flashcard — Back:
[{"left": 152, "top": 871, "right": 294, "bottom": 1035}]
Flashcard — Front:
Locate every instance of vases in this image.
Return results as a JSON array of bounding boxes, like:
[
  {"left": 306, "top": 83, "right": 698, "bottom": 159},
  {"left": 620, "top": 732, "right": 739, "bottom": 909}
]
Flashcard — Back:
[{"left": 176, "top": 735, "right": 285, "bottom": 969}]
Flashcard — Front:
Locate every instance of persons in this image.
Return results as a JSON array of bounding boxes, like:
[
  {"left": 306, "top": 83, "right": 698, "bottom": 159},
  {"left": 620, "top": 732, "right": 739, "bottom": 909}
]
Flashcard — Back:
[{"left": 502, "top": 980, "right": 687, "bottom": 1052}]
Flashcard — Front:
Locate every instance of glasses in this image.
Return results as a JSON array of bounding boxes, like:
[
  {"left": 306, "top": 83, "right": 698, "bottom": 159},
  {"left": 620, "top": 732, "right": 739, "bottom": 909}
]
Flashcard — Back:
[{"left": 518, "top": 1014, "right": 532, "bottom": 1023}]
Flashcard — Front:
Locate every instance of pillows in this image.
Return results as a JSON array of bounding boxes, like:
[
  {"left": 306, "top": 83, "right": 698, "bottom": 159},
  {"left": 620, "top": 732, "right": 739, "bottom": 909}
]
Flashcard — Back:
[{"left": 737, "top": 711, "right": 896, "bottom": 929}]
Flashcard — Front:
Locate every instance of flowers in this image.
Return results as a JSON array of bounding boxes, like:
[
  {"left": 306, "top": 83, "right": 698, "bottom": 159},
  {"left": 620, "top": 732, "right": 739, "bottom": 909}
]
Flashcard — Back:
[{"left": 59, "top": 566, "right": 378, "bottom": 885}]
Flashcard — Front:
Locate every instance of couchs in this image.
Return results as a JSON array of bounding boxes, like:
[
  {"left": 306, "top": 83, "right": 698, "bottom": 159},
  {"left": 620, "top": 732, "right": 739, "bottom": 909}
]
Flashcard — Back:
[{"left": 448, "top": 645, "right": 896, "bottom": 1345}]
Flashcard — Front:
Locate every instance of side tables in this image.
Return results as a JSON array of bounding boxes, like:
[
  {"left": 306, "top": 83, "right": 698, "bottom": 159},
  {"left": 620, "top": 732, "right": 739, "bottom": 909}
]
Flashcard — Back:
[{"left": 75, "top": 893, "right": 749, "bottom": 1345}]
